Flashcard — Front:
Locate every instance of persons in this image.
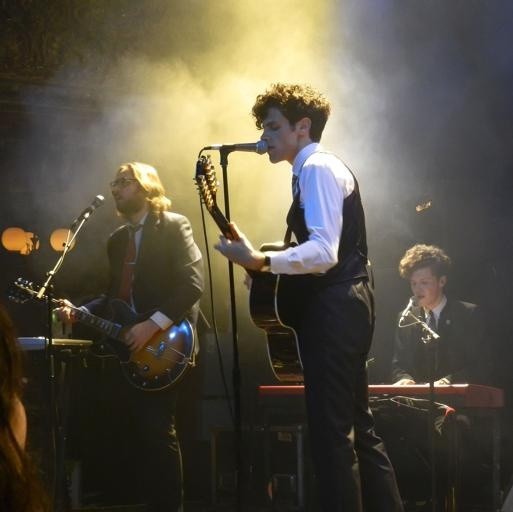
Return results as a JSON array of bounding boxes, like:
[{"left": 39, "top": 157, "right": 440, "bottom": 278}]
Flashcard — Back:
[
  {"left": 56, "top": 162, "right": 210, "bottom": 512},
  {"left": 214, "top": 84, "right": 404, "bottom": 512},
  {"left": 392, "top": 244, "right": 494, "bottom": 436},
  {"left": 0, "top": 306, "right": 27, "bottom": 512}
]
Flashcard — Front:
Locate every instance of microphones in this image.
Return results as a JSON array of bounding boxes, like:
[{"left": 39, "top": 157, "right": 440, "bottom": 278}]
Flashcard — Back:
[
  {"left": 400, "top": 296, "right": 418, "bottom": 324},
  {"left": 205, "top": 140, "right": 268, "bottom": 155},
  {"left": 71, "top": 194, "right": 105, "bottom": 232}
]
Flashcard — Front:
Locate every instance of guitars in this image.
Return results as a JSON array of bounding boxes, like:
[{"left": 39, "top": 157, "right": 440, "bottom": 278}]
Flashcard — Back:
[
  {"left": 193, "top": 155, "right": 304, "bottom": 382},
  {"left": 9, "top": 278, "right": 195, "bottom": 392}
]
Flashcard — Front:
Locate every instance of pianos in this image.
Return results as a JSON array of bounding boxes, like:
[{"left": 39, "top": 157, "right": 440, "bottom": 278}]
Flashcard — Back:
[{"left": 257, "top": 384, "right": 504, "bottom": 408}]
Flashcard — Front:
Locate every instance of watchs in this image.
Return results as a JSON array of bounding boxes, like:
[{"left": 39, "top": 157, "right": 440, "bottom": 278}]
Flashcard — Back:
[{"left": 260, "top": 256, "right": 270, "bottom": 272}]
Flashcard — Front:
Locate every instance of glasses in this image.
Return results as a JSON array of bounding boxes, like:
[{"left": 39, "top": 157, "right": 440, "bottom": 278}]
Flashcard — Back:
[{"left": 110, "top": 178, "right": 137, "bottom": 189}]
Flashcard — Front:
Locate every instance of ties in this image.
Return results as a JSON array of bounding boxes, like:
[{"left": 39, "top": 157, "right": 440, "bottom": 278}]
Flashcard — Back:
[
  {"left": 425, "top": 309, "right": 438, "bottom": 335},
  {"left": 118, "top": 228, "right": 138, "bottom": 308},
  {"left": 291, "top": 172, "right": 298, "bottom": 201}
]
